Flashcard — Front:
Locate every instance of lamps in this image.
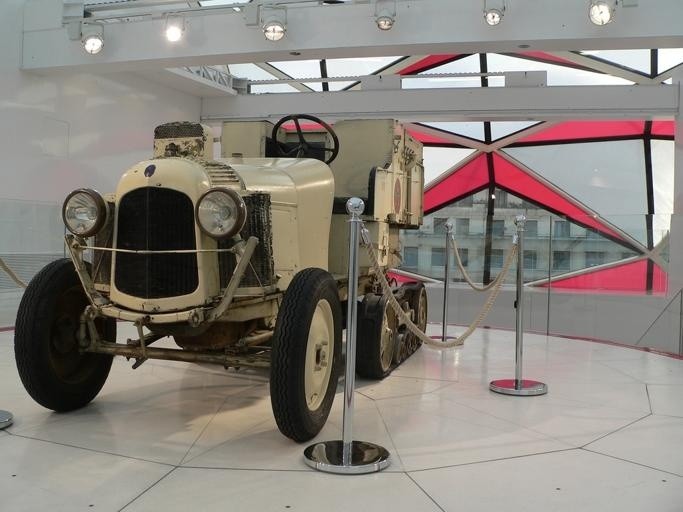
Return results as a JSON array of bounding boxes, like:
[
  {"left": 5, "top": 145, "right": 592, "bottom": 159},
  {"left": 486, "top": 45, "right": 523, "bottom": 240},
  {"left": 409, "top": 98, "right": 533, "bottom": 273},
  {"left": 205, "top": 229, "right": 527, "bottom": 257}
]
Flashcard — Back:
[
  {"left": 369, "top": 1, "right": 401, "bottom": 32},
  {"left": 479, "top": 0, "right": 510, "bottom": 29},
  {"left": 250, "top": 2, "right": 294, "bottom": 42},
  {"left": 583, "top": 0, "right": 617, "bottom": 30},
  {"left": 73, "top": 18, "right": 109, "bottom": 55},
  {"left": 156, "top": 9, "right": 194, "bottom": 46}
]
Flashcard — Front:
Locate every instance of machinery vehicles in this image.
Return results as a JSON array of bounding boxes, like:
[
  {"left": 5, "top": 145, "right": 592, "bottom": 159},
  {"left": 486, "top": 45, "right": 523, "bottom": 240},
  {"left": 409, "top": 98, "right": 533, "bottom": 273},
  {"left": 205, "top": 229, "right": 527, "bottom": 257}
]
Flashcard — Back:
[{"left": 14, "top": 114, "right": 428, "bottom": 442}]
